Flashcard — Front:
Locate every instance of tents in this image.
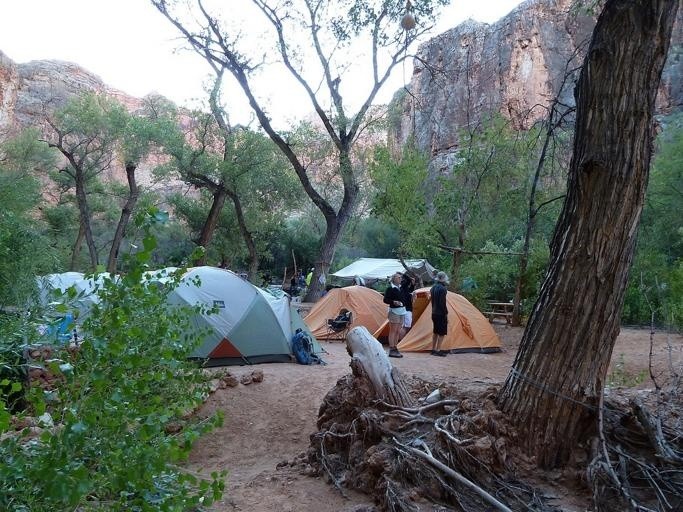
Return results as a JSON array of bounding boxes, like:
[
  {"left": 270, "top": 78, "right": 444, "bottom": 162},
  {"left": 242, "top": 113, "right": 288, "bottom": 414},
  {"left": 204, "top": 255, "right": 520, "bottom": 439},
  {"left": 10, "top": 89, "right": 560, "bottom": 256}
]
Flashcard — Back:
[
  {"left": 142, "top": 266, "right": 329, "bottom": 367},
  {"left": 23, "top": 272, "right": 124, "bottom": 348},
  {"left": 303, "top": 285, "right": 500, "bottom": 353}
]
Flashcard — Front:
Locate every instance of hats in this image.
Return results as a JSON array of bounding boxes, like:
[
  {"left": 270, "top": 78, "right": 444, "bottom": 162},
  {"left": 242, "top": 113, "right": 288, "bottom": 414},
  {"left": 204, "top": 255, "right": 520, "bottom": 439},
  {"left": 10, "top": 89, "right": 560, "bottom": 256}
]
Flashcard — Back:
[{"left": 435, "top": 271, "right": 449, "bottom": 282}]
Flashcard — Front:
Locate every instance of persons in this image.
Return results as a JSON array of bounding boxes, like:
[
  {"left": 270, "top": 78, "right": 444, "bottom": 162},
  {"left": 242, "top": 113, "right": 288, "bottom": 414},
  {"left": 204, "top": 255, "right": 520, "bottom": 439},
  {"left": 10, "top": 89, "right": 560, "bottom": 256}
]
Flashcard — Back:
[
  {"left": 430, "top": 269, "right": 448, "bottom": 357},
  {"left": 383, "top": 274, "right": 415, "bottom": 358},
  {"left": 290, "top": 267, "right": 314, "bottom": 297}
]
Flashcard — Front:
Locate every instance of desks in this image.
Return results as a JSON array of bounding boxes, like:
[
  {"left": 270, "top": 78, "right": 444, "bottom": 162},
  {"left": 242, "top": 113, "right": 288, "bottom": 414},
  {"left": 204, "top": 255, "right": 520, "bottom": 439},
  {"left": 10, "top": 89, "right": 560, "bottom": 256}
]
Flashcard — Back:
[{"left": 487, "top": 302, "right": 522, "bottom": 324}]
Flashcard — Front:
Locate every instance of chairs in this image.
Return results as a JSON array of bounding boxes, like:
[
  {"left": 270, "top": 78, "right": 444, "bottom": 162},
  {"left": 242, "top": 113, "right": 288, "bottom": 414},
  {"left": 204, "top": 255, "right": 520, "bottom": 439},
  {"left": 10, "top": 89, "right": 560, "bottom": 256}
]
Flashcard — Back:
[{"left": 325, "top": 307, "right": 353, "bottom": 342}]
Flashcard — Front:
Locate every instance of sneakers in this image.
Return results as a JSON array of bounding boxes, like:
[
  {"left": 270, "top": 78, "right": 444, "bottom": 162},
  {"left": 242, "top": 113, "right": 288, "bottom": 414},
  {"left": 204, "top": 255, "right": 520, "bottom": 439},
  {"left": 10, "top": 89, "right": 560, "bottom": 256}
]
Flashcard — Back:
[
  {"left": 389, "top": 349, "right": 403, "bottom": 358},
  {"left": 432, "top": 350, "right": 446, "bottom": 356}
]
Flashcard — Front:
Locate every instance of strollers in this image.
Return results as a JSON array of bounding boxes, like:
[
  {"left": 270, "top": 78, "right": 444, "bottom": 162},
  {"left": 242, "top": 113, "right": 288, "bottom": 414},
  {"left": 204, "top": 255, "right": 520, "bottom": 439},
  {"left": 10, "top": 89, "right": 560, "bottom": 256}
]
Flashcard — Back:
[{"left": 323, "top": 308, "right": 352, "bottom": 344}]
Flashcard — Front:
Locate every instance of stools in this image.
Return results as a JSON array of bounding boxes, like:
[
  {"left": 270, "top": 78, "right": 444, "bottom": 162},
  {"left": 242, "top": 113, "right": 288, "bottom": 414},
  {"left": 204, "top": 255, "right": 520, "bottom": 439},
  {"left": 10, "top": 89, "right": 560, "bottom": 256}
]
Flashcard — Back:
[{"left": 483, "top": 311, "right": 513, "bottom": 324}]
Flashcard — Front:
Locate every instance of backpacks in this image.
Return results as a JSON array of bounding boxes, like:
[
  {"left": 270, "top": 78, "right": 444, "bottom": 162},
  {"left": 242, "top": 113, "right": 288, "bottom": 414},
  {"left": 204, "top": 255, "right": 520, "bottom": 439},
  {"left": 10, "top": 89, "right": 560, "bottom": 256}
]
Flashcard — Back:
[
  {"left": 332, "top": 311, "right": 352, "bottom": 328},
  {"left": 293, "top": 328, "right": 314, "bottom": 365}
]
